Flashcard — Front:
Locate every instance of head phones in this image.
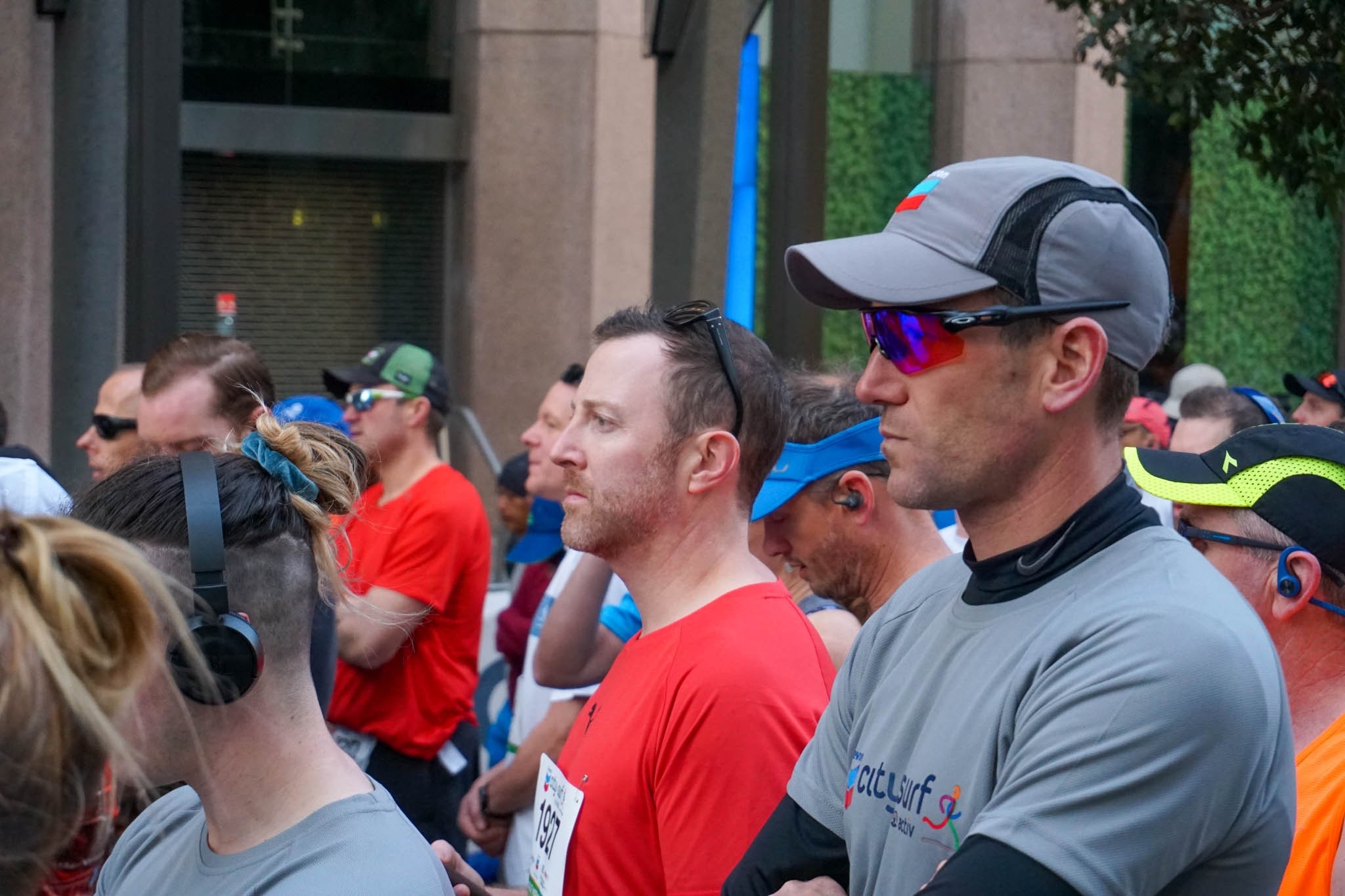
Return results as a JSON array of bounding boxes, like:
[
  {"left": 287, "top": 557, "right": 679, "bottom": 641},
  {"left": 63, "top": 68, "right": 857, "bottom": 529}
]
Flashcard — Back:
[{"left": 165, "top": 449, "right": 262, "bottom": 705}]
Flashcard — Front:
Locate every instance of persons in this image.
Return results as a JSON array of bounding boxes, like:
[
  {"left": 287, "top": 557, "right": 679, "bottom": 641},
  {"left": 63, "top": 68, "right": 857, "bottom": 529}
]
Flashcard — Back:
[
  {"left": 1118, "top": 362, "right": 1345, "bottom": 896},
  {"left": 718, "top": 155, "right": 1294, "bottom": 896},
  {"left": 0, "top": 299, "right": 967, "bottom": 896}
]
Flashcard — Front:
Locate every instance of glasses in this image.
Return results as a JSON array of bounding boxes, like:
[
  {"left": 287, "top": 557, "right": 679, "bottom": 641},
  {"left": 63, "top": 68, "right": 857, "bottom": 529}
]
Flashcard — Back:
[
  {"left": 90, "top": 410, "right": 138, "bottom": 441},
  {"left": 1176, "top": 517, "right": 1345, "bottom": 590},
  {"left": 860, "top": 296, "right": 1134, "bottom": 383},
  {"left": 1311, "top": 367, "right": 1344, "bottom": 402},
  {"left": 561, "top": 363, "right": 584, "bottom": 384},
  {"left": 344, "top": 387, "right": 417, "bottom": 411}
]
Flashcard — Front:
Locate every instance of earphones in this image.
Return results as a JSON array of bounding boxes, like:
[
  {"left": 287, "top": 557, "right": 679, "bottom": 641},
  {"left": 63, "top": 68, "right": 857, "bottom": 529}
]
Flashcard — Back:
[
  {"left": 1277, "top": 545, "right": 1310, "bottom": 598},
  {"left": 846, "top": 494, "right": 861, "bottom": 510}
]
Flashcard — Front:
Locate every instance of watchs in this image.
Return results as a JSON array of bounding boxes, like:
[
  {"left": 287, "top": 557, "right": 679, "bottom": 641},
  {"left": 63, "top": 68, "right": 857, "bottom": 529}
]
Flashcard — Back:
[{"left": 478, "top": 781, "right": 515, "bottom": 821}]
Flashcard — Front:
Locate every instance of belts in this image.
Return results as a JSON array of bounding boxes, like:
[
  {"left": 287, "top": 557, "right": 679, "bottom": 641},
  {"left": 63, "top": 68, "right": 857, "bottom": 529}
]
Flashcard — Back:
[{"left": 437, "top": 738, "right": 466, "bottom": 777}]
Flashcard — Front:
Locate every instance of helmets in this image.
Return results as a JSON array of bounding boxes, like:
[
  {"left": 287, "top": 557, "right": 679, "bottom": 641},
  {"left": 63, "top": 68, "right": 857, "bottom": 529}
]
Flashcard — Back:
[{"left": 1123, "top": 396, "right": 1171, "bottom": 449}]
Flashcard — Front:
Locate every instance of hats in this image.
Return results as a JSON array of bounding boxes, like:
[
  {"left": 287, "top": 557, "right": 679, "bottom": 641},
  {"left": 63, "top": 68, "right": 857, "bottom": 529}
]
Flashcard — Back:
[
  {"left": 778, "top": 152, "right": 1173, "bottom": 373},
  {"left": 320, "top": 340, "right": 453, "bottom": 417},
  {"left": 0, "top": 455, "right": 76, "bottom": 523},
  {"left": 275, "top": 393, "right": 352, "bottom": 438},
  {"left": 662, "top": 298, "right": 744, "bottom": 440},
  {"left": 504, "top": 496, "right": 565, "bottom": 565},
  {"left": 1123, "top": 421, "right": 1345, "bottom": 575},
  {"left": 1283, "top": 368, "right": 1345, "bottom": 408},
  {"left": 1160, "top": 363, "right": 1228, "bottom": 421},
  {"left": 748, "top": 415, "right": 890, "bottom": 525}
]
